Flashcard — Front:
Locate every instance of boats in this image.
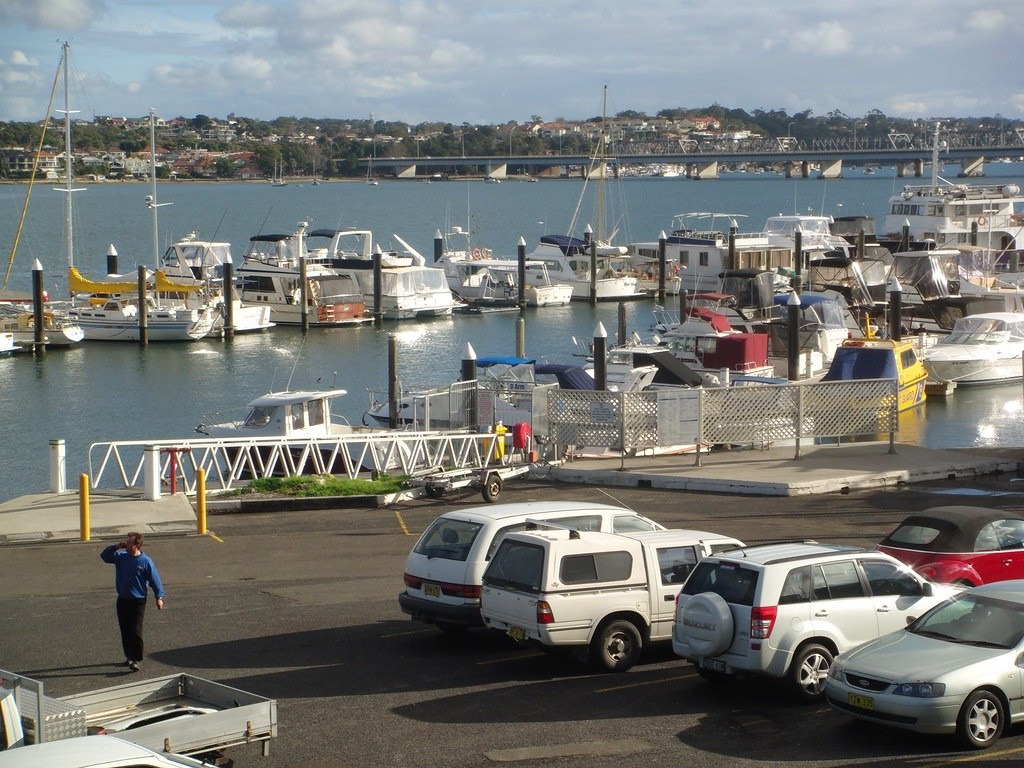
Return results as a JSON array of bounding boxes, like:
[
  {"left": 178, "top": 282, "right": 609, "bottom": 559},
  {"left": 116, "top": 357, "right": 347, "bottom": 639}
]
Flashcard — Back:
[
  {"left": 819, "top": 311, "right": 943, "bottom": 417},
  {"left": 69, "top": 113, "right": 1023, "bottom": 457},
  {"left": 925, "top": 308, "right": 1022, "bottom": 385},
  {"left": 193, "top": 371, "right": 428, "bottom": 479}
]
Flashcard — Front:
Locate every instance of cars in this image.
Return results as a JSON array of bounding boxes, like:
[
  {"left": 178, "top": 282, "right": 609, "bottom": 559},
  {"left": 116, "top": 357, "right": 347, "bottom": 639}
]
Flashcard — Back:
[
  {"left": 869, "top": 504, "right": 1024, "bottom": 590},
  {"left": 0, "top": 735, "right": 226, "bottom": 768},
  {"left": 821, "top": 576, "right": 1024, "bottom": 747}
]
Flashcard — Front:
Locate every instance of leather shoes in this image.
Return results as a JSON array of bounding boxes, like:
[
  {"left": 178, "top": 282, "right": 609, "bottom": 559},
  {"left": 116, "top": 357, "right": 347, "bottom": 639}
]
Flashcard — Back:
[{"left": 122, "top": 659, "right": 139, "bottom": 672}]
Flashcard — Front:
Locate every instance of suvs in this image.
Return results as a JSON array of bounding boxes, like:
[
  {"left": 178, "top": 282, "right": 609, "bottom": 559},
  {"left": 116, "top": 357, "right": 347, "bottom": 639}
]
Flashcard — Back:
[{"left": 670, "top": 538, "right": 970, "bottom": 702}]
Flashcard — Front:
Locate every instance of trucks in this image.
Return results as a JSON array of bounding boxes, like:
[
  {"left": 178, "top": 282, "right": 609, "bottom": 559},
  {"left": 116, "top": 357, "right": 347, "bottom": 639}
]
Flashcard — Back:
[{"left": 1, "top": 665, "right": 279, "bottom": 768}]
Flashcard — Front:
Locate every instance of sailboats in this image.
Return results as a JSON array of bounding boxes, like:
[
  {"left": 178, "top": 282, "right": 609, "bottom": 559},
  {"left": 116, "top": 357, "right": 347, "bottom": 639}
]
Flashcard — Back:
[
  {"left": 524, "top": 79, "right": 639, "bottom": 308},
  {"left": 0, "top": 38, "right": 85, "bottom": 356}
]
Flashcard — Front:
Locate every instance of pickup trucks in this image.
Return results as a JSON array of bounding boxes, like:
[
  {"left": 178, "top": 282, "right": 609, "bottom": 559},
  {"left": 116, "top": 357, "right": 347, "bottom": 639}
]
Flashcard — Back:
[{"left": 480, "top": 524, "right": 748, "bottom": 675}]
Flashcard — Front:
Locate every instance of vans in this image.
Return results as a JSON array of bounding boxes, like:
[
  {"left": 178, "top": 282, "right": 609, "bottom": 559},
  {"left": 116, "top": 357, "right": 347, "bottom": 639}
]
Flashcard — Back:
[{"left": 395, "top": 487, "right": 668, "bottom": 640}]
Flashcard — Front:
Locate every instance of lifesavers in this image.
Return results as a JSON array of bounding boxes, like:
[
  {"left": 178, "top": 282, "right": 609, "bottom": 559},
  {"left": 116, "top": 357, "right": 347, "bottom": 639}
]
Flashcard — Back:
[
  {"left": 978, "top": 216, "right": 985, "bottom": 226},
  {"left": 472, "top": 248, "right": 481, "bottom": 260},
  {"left": 481, "top": 248, "right": 489, "bottom": 259}
]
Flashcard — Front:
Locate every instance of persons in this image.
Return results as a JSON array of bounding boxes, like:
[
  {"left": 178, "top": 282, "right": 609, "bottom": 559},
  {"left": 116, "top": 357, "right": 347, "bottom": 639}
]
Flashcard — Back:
[{"left": 100, "top": 532, "right": 163, "bottom": 671}]
